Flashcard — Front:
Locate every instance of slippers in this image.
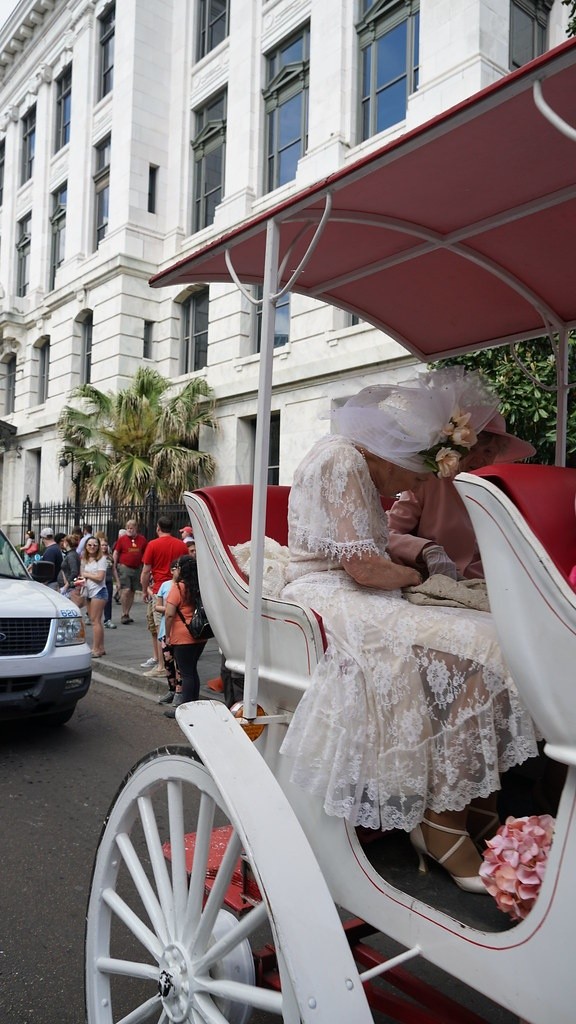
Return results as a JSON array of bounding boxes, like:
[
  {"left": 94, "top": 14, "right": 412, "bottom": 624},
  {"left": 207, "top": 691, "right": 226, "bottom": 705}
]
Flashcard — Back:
[
  {"left": 101, "top": 649, "right": 105, "bottom": 655},
  {"left": 91, "top": 649, "right": 101, "bottom": 657}
]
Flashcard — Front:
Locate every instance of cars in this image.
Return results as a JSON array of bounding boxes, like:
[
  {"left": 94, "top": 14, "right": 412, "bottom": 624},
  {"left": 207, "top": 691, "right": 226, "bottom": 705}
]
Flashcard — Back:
[{"left": 0, "top": 531, "right": 93, "bottom": 727}]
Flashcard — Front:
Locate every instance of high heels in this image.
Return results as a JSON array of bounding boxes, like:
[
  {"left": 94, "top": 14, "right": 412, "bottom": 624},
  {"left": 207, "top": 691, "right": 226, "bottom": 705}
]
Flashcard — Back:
[
  {"left": 469, "top": 806, "right": 504, "bottom": 858},
  {"left": 410, "top": 816, "right": 491, "bottom": 894}
]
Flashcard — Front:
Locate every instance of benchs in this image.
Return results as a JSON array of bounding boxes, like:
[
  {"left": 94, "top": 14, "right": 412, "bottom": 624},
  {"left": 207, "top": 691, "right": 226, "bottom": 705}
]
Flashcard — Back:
[
  {"left": 453, "top": 462, "right": 576, "bottom": 749},
  {"left": 182, "top": 486, "right": 326, "bottom": 679}
]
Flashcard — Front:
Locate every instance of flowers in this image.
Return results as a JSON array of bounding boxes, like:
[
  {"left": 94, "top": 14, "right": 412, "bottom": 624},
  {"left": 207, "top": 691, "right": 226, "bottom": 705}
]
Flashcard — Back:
[{"left": 422, "top": 411, "right": 479, "bottom": 480}]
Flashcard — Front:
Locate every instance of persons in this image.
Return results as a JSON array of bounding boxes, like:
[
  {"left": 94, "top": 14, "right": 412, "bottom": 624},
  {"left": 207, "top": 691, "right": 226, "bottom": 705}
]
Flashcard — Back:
[
  {"left": 228, "top": 365, "right": 544, "bottom": 892},
  {"left": 20, "top": 516, "right": 215, "bottom": 719}
]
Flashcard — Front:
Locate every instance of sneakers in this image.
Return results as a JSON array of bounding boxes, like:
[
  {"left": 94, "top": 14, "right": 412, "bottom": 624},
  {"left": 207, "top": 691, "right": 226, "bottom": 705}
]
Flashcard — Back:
[
  {"left": 140, "top": 657, "right": 159, "bottom": 668},
  {"left": 143, "top": 665, "right": 167, "bottom": 677}
]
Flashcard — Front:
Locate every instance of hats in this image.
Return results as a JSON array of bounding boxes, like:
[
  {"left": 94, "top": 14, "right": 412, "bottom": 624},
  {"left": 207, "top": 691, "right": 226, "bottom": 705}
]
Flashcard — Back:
[
  {"left": 317, "top": 364, "right": 503, "bottom": 479},
  {"left": 39, "top": 528, "right": 54, "bottom": 539},
  {"left": 180, "top": 526, "right": 192, "bottom": 535},
  {"left": 457, "top": 405, "right": 536, "bottom": 465}
]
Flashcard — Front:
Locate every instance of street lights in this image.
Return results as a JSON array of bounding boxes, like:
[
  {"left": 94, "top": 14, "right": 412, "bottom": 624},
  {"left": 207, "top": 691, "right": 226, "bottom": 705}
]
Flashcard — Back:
[{"left": 60, "top": 450, "right": 81, "bottom": 529}]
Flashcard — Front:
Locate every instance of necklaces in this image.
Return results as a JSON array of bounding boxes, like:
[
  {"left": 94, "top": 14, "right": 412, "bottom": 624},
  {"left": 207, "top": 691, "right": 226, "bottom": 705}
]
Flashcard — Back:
[{"left": 361, "top": 449, "right": 366, "bottom": 460}]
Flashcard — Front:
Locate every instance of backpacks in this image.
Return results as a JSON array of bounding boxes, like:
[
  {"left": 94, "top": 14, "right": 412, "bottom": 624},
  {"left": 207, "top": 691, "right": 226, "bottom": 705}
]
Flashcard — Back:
[{"left": 176, "top": 597, "right": 214, "bottom": 640}]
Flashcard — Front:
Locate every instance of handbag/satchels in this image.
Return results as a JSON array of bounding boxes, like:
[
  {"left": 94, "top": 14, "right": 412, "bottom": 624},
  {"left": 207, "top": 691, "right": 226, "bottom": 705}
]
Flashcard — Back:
[{"left": 24, "top": 538, "right": 39, "bottom": 555}]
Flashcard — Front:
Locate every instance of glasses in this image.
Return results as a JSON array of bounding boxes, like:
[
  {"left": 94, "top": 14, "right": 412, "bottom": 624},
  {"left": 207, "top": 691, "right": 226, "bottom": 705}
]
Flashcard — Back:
[{"left": 87, "top": 544, "right": 99, "bottom": 547}]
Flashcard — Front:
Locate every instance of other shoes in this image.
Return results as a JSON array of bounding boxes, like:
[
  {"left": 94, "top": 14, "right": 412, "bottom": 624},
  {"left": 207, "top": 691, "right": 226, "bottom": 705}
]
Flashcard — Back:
[
  {"left": 165, "top": 692, "right": 184, "bottom": 718},
  {"left": 103, "top": 620, "right": 117, "bottom": 629},
  {"left": 159, "top": 689, "right": 174, "bottom": 702},
  {"left": 207, "top": 678, "right": 226, "bottom": 692},
  {"left": 84, "top": 615, "right": 91, "bottom": 625},
  {"left": 121, "top": 615, "right": 134, "bottom": 624}
]
